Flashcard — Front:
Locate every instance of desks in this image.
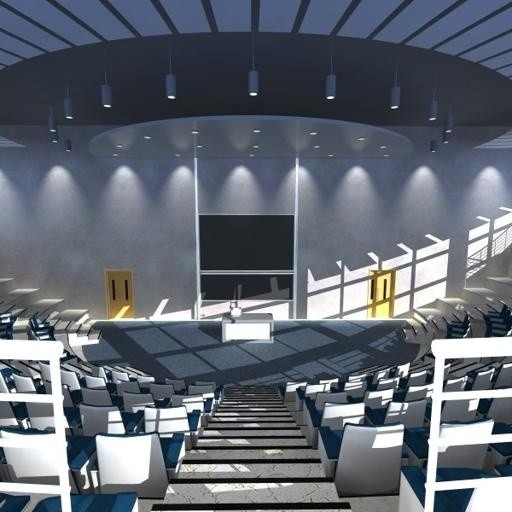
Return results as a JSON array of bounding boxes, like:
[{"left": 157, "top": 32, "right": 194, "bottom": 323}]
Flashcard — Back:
[{"left": 222, "top": 312, "right": 274, "bottom": 345}]
